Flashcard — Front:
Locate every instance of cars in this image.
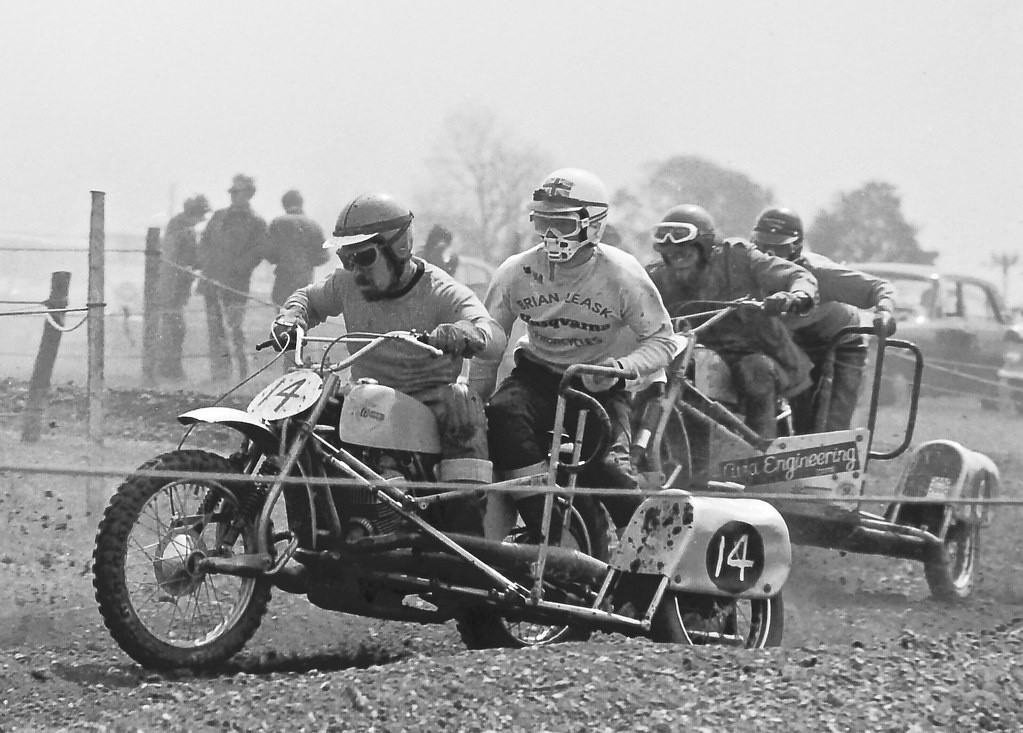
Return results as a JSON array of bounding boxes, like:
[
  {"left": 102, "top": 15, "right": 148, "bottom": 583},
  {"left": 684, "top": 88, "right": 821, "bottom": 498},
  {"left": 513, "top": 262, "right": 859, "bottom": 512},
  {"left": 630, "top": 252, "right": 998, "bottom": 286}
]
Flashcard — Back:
[
  {"left": 842, "top": 264, "right": 1023, "bottom": 408},
  {"left": 445, "top": 256, "right": 496, "bottom": 304}
]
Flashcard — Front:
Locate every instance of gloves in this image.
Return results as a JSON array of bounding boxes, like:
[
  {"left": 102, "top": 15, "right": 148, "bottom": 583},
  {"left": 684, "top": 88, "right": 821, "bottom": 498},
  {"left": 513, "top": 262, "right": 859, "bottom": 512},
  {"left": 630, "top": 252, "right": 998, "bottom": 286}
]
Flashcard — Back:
[
  {"left": 765, "top": 290, "right": 812, "bottom": 313},
  {"left": 872, "top": 309, "right": 897, "bottom": 337},
  {"left": 428, "top": 319, "right": 486, "bottom": 360},
  {"left": 270, "top": 308, "right": 309, "bottom": 352}
]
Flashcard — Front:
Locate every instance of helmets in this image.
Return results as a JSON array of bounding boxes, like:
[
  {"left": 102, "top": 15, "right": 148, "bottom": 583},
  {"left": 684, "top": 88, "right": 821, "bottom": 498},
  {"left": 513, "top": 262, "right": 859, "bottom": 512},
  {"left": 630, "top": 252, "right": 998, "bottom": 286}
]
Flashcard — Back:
[
  {"left": 752, "top": 206, "right": 804, "bottom": 263},
  {"left": 322, "top": 190, "right": 414, "bottom": 262},
  {"left": 525, "top": 168, "right": 611, "bottom": 262},
  {"left": 652, "top": 204, "right": 714, "bottom": 260}
]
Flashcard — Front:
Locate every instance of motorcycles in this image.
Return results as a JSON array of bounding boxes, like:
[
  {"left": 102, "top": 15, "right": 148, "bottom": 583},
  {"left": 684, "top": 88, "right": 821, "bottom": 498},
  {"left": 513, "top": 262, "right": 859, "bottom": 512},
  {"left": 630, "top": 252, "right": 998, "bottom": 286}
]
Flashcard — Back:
[
  {"left": 92, "top": 319, "right": 793, "bottom": 677},
  {"left": 620, "top": 294, "right": 1000, "bottom": 605}
]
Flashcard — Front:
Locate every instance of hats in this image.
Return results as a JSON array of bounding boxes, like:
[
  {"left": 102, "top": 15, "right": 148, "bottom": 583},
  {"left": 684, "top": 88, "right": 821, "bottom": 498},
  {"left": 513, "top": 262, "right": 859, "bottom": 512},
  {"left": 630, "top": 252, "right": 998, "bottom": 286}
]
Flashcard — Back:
[
  {"left": 184, "top": 195, "right": 213, "bottom": 217},
  {"left": 228, "top": 174, "right": 253, "bottom": 191}
]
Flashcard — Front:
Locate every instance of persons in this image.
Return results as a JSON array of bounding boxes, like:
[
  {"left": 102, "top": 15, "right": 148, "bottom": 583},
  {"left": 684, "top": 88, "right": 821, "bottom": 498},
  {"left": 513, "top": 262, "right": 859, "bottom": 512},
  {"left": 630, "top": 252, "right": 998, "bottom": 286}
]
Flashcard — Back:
[
  {"left": 158, "top": 175, "right": 329, "bottom": 388},
  {"left": 600, "top": 201, "right": 899, "bottom": 450},
  {"left": 414, "top": 223, "right": 459, "bottom": 279},
  {"left": 271, "top": 193, "right": 508, "bottom": 540},
  {"left": 469, "top": 169, "right": 677, "bottom": 551}
]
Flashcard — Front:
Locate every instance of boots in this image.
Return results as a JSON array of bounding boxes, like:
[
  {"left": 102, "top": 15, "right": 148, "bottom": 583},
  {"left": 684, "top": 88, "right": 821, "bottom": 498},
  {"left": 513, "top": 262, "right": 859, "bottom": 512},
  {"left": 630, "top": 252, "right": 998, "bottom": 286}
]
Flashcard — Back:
[
  {"left": 433, "top": 457, "right": 493, "bottom": 555},
  {"left": 502, "top": 460, "right": 581, "bottom": 557},
  {"left": 781, "top": 377, "right": 816, "bottom": 443},
  {"left": 744, "top": 387, "right": 777, "bottom": 454}
]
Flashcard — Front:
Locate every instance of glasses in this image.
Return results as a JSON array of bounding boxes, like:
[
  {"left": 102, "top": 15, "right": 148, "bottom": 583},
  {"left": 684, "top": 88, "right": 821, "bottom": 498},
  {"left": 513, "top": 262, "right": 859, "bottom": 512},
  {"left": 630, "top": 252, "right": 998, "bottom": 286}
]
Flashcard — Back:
[
  {"left": 336, "top": 242, "right": 382, "bottom": 272},
  {"left": 529, "top": 210, "right": 589, "bottom": 239},
  {"left": 650, "top": 222, "right": 698, "bottom": 244}
]
